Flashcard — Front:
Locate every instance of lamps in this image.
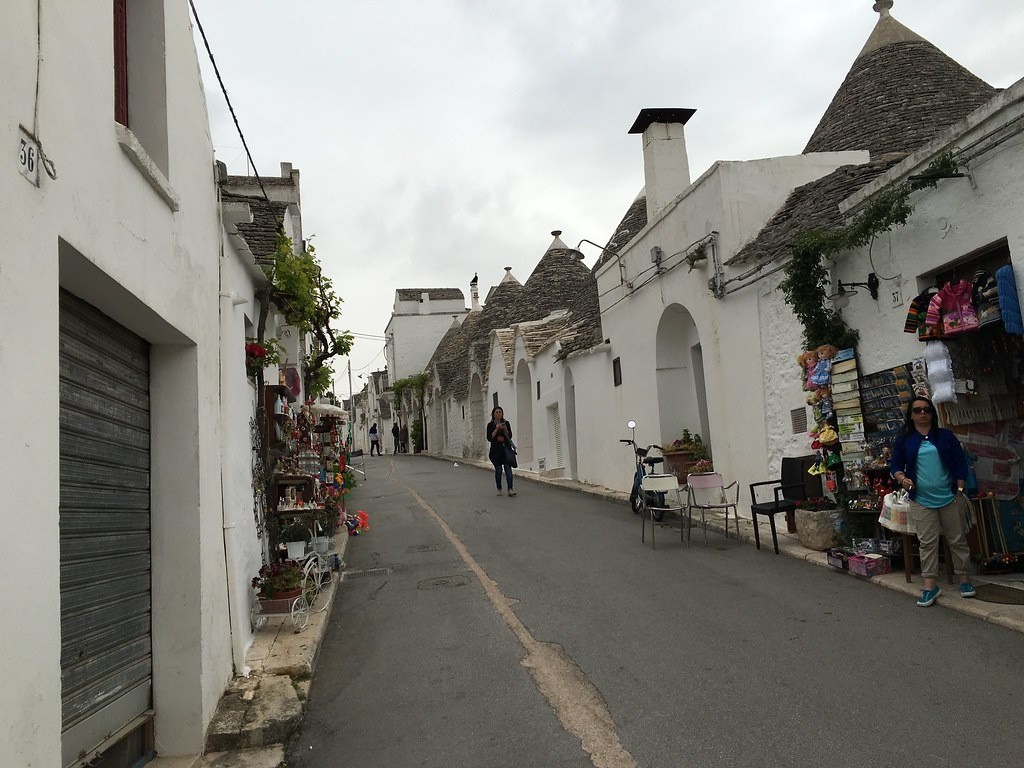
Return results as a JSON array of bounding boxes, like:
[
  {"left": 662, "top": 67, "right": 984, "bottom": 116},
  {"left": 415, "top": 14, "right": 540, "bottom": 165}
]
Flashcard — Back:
[
  {"left": 826, "top": 272, "right": 879, "bottom": 308},
  {"left": 566, "top": 239, "right": 626, "bottom": 286}
]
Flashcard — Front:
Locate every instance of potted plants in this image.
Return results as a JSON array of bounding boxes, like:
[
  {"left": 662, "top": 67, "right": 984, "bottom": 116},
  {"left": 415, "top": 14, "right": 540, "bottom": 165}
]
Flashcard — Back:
[
  {"left": 279, "top": 522, "right": 313, "bottom": 560},
  {"left": 685, "top": 445, "right": 712, "bottom": 470},
  {"left": 311, "top": 515, "right": 333, "bottom": 555}
]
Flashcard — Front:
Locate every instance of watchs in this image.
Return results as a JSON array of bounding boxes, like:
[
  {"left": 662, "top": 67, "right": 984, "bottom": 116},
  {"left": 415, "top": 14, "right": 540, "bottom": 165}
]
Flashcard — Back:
[{"left": 957, "top": 487, "right": 965, "bottom": 492}]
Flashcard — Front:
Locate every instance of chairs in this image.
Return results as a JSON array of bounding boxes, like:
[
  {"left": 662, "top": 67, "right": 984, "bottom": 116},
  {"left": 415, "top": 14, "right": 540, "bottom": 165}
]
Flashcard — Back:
[
  {"left": 685, "top": 472, "right": 741, "bottom": 550},
  {"left": 349, "top": 449, "right": 367, "bottom": 481},
  {"left": 749, "top": 457, "right": 808, "bottom": 555},
  {"left": 639, "top": 473, "right": 690, "bottom": 550}
]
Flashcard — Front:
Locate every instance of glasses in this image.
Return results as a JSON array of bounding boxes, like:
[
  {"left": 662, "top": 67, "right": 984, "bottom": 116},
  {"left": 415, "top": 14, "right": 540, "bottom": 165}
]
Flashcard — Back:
[{"left": 911, "top": 406, "right": 931, "bottom": 415}]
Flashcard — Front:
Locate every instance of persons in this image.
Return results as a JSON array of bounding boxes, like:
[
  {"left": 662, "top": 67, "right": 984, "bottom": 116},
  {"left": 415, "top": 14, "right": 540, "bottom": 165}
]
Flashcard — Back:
[
  {"left": 339, "top": 440, "right": 343, "bottom": 449},
  {"left": 890, "top": 397, "right": 976, "bottom": 606},
  {"left": 345, "top": 437, "right": 350, "bottom": 448},
  {"left": 369, "top": 423, "right": 383, "bottom": 456},
  {"left": 392, "top": 422, "right": 408, "bottom": 454},
  {"left": 486, "top": 406, "right": 518, "bottom": 496}
]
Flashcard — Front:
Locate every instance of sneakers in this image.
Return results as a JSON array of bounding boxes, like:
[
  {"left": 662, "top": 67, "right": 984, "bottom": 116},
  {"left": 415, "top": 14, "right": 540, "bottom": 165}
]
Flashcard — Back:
[
  {"left": 961, "top": 580, "right": 976, "bottom": 597},
  {"left": 916, "top": 585, "right": 942, "bottom": 607}
]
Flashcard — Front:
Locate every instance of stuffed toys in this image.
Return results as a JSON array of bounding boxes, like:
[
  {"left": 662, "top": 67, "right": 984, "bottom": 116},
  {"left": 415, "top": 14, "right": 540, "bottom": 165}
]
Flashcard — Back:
[{"left": 796, "top": 344, "right": 840, "bottom": 476}]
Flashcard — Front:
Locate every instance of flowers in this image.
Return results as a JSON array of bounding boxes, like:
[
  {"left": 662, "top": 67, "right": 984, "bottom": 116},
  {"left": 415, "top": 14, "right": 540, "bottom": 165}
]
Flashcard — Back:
[
  {"left": 660, "top": 428, "right": 706, "bottom": 453},
  {"left": 245, "top": 336, "right": 287, "bottom": 371},
  {"left": 684, "top": 459, "right": 713, "bottom": 474},
  {"left": 251, "top": 560, "right": 306, "bottom": 601},
  {"left": 793, "top": 495, "right": 837, "bottom": 512}
]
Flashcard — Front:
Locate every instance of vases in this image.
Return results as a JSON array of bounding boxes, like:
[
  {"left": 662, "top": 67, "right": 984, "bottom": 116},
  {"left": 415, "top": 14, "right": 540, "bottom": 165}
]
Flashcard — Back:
[
  {"left": 256, "top": 588, "right": 302, "bottom": 614},
  {"left": 663, "top": 449, "right": 708, "bottom": 485},
  {"left": 247, "top": 364, "right": 261, "bottom": 382},
  {"left": 794, "top": 507, "right": 848, "bottom": 551}
]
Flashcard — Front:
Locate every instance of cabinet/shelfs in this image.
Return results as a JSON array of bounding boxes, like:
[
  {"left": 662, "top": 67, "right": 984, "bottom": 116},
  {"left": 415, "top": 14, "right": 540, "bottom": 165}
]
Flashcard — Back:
[
  {"left": 265, "top": 384, "right": 296, "bottom": 450},
  {"left": 847, "top": 465, "right": 890, "bottom": 538},
  {"left": 274, "top": 484, "right": 314, "bottom": 520}
]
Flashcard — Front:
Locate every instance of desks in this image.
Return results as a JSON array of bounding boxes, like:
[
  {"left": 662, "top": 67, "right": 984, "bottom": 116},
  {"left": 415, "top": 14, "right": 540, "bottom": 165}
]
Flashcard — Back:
[{"left": 878, "top": 493, "right": 978, "bottom": 584}]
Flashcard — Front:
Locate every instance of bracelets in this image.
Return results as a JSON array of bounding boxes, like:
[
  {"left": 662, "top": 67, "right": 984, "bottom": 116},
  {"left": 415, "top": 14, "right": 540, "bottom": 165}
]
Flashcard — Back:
[{"left": 901, "top": 477, "right": 906, "bottom": 484}]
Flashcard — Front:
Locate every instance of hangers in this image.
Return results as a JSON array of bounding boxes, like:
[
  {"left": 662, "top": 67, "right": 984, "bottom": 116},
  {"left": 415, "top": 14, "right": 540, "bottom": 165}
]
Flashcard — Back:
[
  {"left": 928, "top": 280, "right": 940, "bottom": 294},
  {"left": 949, "top": 270, "right": 960, "bottom": 286}
]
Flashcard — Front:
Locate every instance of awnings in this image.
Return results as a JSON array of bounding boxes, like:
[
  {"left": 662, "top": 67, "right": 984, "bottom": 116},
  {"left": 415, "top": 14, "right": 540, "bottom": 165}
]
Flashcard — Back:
[{"left": 312, "top": 403, "right": 351, "bottom": 415}]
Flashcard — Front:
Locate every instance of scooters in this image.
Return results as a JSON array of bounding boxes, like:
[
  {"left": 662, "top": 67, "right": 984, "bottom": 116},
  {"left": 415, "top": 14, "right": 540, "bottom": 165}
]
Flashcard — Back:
[{"left": 619, "top": 419, "right": 670, "bottom": 522}]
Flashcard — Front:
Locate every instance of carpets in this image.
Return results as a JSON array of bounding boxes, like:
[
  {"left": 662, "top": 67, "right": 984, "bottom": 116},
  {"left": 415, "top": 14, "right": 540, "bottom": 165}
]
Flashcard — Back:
[{"left": 974, "top": 583, "right": 1024, "bottom": 605}]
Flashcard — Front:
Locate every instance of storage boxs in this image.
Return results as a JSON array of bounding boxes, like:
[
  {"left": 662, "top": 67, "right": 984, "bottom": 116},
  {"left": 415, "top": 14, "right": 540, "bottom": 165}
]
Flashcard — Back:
[
  {"left": 851, "top": 538, "right": 880, "bottom": 550},
  {"left": 826, "top": 548, "right": 856, "bottom": 570},
  {"left": 848, "top": 556, "right": 893, "bottom": 578}
]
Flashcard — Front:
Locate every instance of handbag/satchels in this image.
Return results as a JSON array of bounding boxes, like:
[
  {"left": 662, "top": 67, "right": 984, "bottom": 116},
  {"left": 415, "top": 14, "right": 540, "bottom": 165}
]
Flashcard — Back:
[{"left": 511, "top": 450, "right": 518, "bottom": 469}]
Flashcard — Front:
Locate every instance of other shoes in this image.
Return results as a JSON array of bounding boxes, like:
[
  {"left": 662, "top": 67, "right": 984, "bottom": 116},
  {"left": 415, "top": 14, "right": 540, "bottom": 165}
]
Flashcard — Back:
[
  {"left": 508, "top": 491, "right": 516, "bottom": 496},
  {"left": 496, "top": 488, "right": 502, "bottom": 496}
]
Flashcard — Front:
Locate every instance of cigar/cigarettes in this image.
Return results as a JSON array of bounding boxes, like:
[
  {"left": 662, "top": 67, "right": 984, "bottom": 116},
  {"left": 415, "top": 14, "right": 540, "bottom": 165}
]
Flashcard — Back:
[{"left": 909, "top": 486, "right": 912, "bottom": 489}]
